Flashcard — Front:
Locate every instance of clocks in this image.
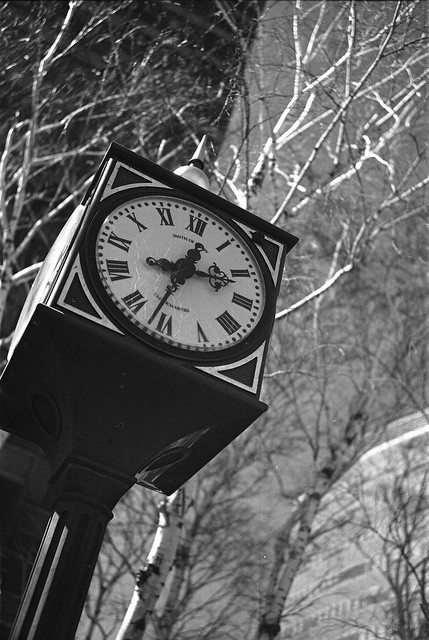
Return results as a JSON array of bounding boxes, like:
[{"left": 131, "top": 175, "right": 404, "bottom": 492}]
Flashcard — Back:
[{"left": 0, "top": 134, "right": 299, "bottom": 496}]
